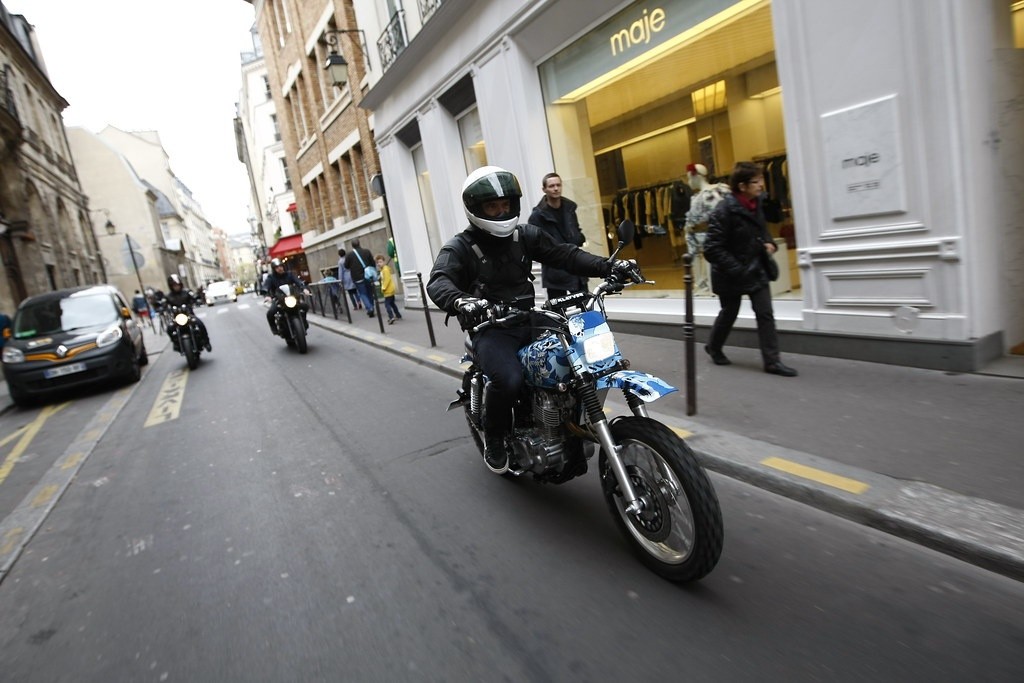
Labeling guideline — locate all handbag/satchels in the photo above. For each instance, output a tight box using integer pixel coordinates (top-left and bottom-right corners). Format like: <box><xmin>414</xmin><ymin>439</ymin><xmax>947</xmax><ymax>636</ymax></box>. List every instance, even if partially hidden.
<box><xmin>364</xmin><ymin>266</ymin><xmax>377</xmax><ymax>282</ymax></box>
<box><xmin>768</xmin><ymin>257</ymin><xmax>778</xmax><ymax>281</ymax></box>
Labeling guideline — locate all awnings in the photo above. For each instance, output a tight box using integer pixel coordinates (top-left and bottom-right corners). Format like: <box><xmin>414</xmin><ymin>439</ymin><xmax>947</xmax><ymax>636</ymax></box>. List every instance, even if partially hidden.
<box><xmin>267</xmin><ymin>233</ymin><xmax>304</xmax><ymax>260</ymax></box>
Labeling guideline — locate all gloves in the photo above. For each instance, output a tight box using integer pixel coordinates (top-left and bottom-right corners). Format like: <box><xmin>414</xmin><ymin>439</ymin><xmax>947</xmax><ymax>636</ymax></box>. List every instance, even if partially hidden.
<box><xmin>454</xmin><ymin>297</ymin><xmax>496</xmax><ymax>329</ymax></box>
<box><xmin>610</xmin><ymin>258</ymin><xmax>647</xmax><ymax>285</ymax></box>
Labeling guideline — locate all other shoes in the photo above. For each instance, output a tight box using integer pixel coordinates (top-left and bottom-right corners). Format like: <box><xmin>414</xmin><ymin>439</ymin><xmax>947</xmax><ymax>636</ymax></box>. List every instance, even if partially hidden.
<box><xmin>359</xmin><ymin>304</ymin><xmax>362</xmax><ymax>309</ymax></box>
<box><xmin>205</xmin><ymin>343</ymin><xmax>211</xmax><ymax>351</ymax></box>
<box><xmin>395</xmin><ymin>317</ymin><xmax>401</xmax><ymax>321</ymax></box>
<box><xmin>367</xmin><ymin>311</ymin><xmax>374</xmax><ymax>317</ymax></box>
<box><xmin>704</xmin><ymin>345</ymin><xmax>731</xmax><ymax>365</ymax></box>
<box><xmin>388</xmin><ymin>317</ymin><xmax>395</xmax><ymax>324</ymax></box>
<box><xmin>174</xmin><ymin>344</ymin><xmax>179</xmax><ymax>351</ymax></box>
<box><xmin>354</xmin><ymin>307</ymin><xmax>357</xmax><ymax>310</ymax></box>
<box><xmin>763</xmin><ymin>360</ymin><xmax>798</xmax><ymax>377</ymax></box>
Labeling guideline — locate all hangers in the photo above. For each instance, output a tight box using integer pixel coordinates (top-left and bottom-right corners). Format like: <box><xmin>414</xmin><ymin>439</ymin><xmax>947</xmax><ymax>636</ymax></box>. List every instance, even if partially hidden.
<box><xmin>612</xmin><ymin>178</ymin><xmax>684</xmax><ymax>199</ymax></box>
<box><xmin>753</xmin><ymin>147</ymin><xmax>787</xmax><ymax>164</ymax></box>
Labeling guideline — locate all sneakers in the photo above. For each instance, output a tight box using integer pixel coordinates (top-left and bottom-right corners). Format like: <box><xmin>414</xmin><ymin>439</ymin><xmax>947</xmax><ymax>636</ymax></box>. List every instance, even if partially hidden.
<box><xmin>483</xmin><ymin>432</ymin><xmax>509</xmax><ymax>474</ymax></box>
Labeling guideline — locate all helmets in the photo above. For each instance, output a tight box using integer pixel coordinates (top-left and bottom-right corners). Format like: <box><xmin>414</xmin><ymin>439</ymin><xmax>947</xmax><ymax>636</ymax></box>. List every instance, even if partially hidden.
<box><xmin>167</xmin><ymin>274</ymin><xmax>183</xmax><ymax>292</ymax></box>
<box><xmin>271</xmin><ymin>258</ymin><xmax>285</xmax><ymax>275</ymax></box>
<box><xmin>461</xmin><ymin>166</ymin><xmax>523</xmax><ymax>237</ymax></box>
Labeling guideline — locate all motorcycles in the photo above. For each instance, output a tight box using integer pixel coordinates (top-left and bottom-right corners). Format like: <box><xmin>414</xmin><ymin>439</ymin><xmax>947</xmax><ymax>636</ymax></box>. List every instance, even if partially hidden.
<box><xmin>152</xmin><ymin>290</ymin><xmax>205</xmax><ymax>370</ymax></box>
<box><xmin>203</xmin><ymin>280</ymin><xmax>238</xmax><ymax>308</ymax></box>
<box><xmin>259</xmin><ymin>275</ymin><xmax>313</xmax><ymax>354</ymax></box>
<box><xmin>444</xmin><ymin>219</ymin><xmax>725</xmax><ymax>586</ymax></box>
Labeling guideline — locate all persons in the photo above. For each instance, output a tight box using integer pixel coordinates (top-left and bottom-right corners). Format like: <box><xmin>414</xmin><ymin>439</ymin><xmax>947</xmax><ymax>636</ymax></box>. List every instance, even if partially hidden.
<box><xmin>261</xmin><ymin>258</ymin><xmax>309</xmax><ymax>335</ymax></box>
<box><xmin>338</xmin><ymin>248</ymin><xmax>362</xmax><ymax>310</ymax></box>
<box><xmin>374</xmin><ymin>254</ymin><xmax>402</xmax><ymax>324</ymax></box>
<box><xmin>527</xmin><ymin>173</ymin><xmax>589</xmax><ymax>317</ymax></box>
<box><xmin>298</xmin><ymin>265</ymin><xmax>312</xmax><ymax>286</ymax></box>
<box><xmin>159</xmin><ymin>274</ymin><xmax>211</xmax><ymax>352</ymax></box>
<box><xmin>323</xmin><ymin>269</ymin><xmax>343</xmax><ymax>314</ymax></box>
<box><xmin>344</xmin><ymin>238</ymin><xmax>375</xmax><ymax>318</ymax></box>
<box><xmin>703</xmin><ymin>162</ymin><xmax>798</xmax><ymax>378</ymax></box>
<box><xmin>427</xmin><ymin>166</ymin><xmax>646</xmax><ymax>473</ymax></box>
<box><xmin>684</xmin><ymin>163</ymin><xmax>731</xmax><ymax>254</ymax></box>
<box><xmin>131</xmin><ymin>285</ymin><xmax>154</xmax><ymax>330</ymax></box>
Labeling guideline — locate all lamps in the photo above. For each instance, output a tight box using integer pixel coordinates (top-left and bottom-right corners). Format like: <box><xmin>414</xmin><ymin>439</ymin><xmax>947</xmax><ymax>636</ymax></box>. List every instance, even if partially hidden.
<box><xmin>91</xmin><ymin>208</ymin><xmax>115</xmax><ymax>236</ymax></box>
<box><xmin>322</xmin><ymin>30</ymin><xmax>372</xmax><ymax>90</ymax></box>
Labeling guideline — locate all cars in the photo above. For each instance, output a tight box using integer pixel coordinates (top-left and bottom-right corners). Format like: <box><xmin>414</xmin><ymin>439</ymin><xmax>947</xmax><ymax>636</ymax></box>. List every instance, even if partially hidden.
<box><xmin>230</xmin><ymin>279</ymin><xmax>244</xmax><ymax>295</ymax></box>
<box><xmin>0</xmin><ymin>284</ymin><xmax>149</xmax><ymax>411</ymax></box>
<box><xmin>243</xmin><ymin>279</ymin><xmax>256</xmax><ymax>294</ymax></box>
